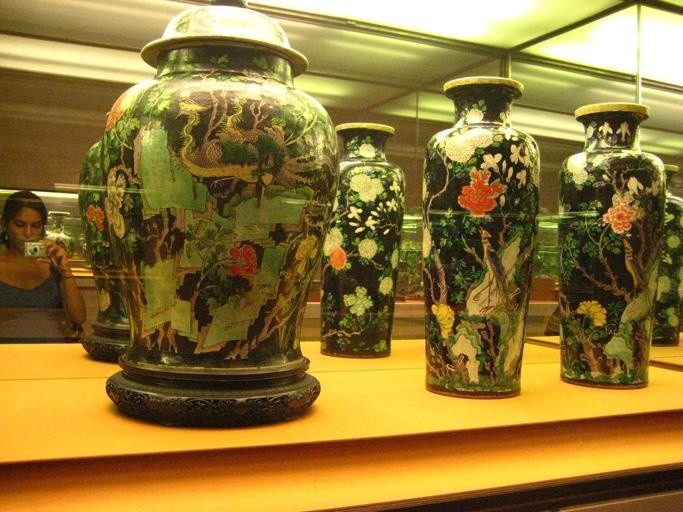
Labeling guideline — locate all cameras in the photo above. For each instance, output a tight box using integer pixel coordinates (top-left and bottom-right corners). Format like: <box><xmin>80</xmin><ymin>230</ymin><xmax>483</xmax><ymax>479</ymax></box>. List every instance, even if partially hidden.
<box><xmin>23</xmin><ymin>241</ymin><xmax>49</xmax><ymax>259</ymax></box>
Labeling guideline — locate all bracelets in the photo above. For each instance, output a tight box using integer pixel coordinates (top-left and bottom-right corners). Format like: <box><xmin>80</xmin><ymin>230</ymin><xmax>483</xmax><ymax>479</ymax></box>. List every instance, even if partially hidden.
<box><xmin>61</xmin><ymin>276</ymin><xmax>74</xmax><ymax>280</ymax></box>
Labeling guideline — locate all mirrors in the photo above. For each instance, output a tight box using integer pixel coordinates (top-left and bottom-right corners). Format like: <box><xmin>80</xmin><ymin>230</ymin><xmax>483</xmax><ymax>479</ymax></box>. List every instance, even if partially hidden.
<box><xmin>508</xmin><ymin>52</ymin><xmax>683</xmax><ymax>373</ymax></box>
<box><xmin>0</xmin><ymin>0</ymin><xmax>507</xmax><ymax>381</ymax></box>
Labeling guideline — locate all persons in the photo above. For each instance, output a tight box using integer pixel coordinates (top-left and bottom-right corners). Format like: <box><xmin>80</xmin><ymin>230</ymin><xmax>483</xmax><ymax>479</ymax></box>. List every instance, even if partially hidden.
<box><xmin>0</xmin><ymin>191</ymin><xmax>87</xmax><ymax>343</ymax></box>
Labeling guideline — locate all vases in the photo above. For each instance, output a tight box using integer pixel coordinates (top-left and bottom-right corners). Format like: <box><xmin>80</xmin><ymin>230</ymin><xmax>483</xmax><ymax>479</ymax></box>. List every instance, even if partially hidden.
<box><xmin>421</xmin><ymin>76</ymin><xmax>540</xmax><ymax>399</ymax></box>
<box><xmin>321</xmin><ymin>122</ymin><xmax>405</xmax><ymax>359</ymax></box>
<box><xmin>652</xmin><ymin>164</ymin><xmax>683</xmax><ymax>347</ymax></box>
<box><xmin>557</xmin><ymin>104</ymin><xmax>667</xmax><ymax>389</ymax></box>
